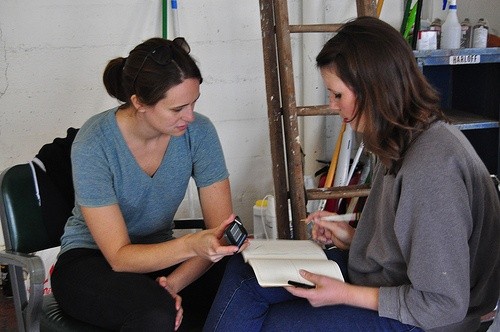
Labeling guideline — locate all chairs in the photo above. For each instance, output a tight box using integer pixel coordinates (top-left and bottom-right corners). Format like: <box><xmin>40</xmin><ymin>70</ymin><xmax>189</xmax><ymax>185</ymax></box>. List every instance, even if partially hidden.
<box><xmin>0</xmin><ymin>127</ymin><xmax>233</xmax><ymax>332</ymax></box>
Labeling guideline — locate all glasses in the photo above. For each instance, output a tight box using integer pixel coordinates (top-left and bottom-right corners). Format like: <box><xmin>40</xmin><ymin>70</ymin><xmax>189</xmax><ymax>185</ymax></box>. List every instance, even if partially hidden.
<box><xmin>132</xmin><ymin>37</ymin><xmax>190</xmax><ymax>96</ymax></box>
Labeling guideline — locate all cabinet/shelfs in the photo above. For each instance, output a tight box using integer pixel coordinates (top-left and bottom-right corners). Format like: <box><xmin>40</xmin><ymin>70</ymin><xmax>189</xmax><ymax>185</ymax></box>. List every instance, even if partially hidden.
<box><xmin>413</xmin><ymin>46</ymin><xmax>500</xmax><ymax>192</ymax></box>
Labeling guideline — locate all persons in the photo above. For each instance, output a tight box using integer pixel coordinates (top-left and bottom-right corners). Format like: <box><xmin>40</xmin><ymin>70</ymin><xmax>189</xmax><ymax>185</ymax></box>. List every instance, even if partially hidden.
<box><xmin>51</xmin><ymin>37</ymin><xmax>250</xmax><ymax>332</ymax></box>
<box><xmin>203</xmin><ymin>15</ymin><xmax>500</xmax><ymax>332</ymax></box>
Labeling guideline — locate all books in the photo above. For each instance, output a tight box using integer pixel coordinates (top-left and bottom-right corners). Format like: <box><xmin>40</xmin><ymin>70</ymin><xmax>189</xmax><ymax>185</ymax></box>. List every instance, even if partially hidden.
<box><xmin>241</xmin><ymin>239</ymin><xmax>344</xmax><ymax>287</ymax></box>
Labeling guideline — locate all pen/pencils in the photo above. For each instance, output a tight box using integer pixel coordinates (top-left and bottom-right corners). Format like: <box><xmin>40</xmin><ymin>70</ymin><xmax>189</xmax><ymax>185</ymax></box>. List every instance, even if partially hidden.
<box><xmin>300</xmin><ymin>213</ymin><xmax>361</xmax><ymax>221</ymax></box>
<box><xmin>288</xmin><ymin>280</ymin><xmax>315</xmax><ymax>289</ymax></box>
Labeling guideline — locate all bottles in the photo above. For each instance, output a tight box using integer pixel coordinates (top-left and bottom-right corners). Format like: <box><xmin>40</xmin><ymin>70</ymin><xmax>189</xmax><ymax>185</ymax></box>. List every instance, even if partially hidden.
<box><xmin>253</xmin><ymin>200</ymin><xmax>268</xmax><ymax>239</ymax></box>
<box><xmin>461</xmin><ymin>18</ymin><xmax>472</xmax><ymax>48</ymax></box>
<box><xmin>472</xmin><ymin>18</ymin><xmax>488</xmax><ymax>48</ymax></box>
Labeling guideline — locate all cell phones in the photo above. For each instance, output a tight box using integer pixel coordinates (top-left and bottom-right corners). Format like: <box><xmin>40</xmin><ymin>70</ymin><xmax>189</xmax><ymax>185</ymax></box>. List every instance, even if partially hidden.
<box><xmin>224</xmin><ymin>216</ymin><xmax>247</xmax><ymax>249</ymax></box>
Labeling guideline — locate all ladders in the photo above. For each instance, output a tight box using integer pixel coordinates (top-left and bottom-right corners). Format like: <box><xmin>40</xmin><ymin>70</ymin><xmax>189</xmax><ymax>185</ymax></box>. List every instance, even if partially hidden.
<box><xmin>261</xmin><ymin>1</ymin><xmax>377</xmax><ymax>240</ymax></box>
